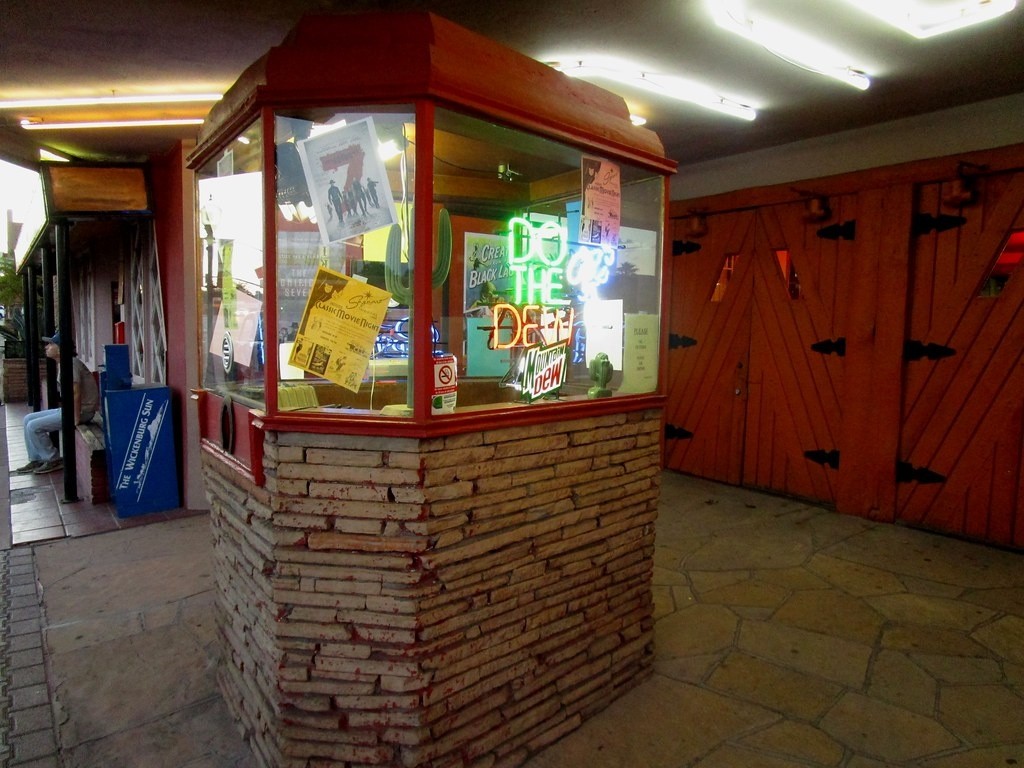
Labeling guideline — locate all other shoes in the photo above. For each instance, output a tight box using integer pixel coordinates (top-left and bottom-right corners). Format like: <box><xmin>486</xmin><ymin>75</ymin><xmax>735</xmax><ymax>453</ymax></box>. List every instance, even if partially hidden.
<box><xmin>329</xmin><ymin>204</ymin><xmax>381</xmax><ymax>222</ymax></box>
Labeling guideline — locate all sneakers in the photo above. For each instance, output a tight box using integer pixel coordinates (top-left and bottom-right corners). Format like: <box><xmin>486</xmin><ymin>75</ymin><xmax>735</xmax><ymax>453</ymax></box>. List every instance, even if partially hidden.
<box><xmin>17</xmin><ymin>457</ymin><xmax>66</xmax><ymax>474</ymax></box>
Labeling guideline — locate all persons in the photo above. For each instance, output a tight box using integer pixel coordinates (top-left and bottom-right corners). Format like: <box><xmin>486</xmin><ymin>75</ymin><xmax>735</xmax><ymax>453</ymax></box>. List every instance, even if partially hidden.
<box><xmin>469</xmin><ymin>247</ymin><xmax>492</xmax><ymax>269</ymax></box>
<box><xmin>14</xmin><ymin>331</ymin><xmax>99</xmax><ymax>475</ymax></box>
<box><xmin>289</xmin><ymin>321</ymin><xmax>301</xmax><ymax>342</ymax></box>
<box><xmin>328</xmin><ymin>180</ymin><xmax>344</xmax><ymax>223</ymax></box>
<box><xmin>470</xmin><ymin>283</ymin><xmax>511</xmax><ymax>310</ymax></box>
<box><xmin>279</xmin><ymin>327</ymin><xmax>288</xmax><ymax>342</ymax></box>
<box><xmin>341</xmin><ymin>177</ymin><xmax>375</xmax><ymax>217</ymax></box>
<box><xmin>367</xmin><ymin>177</ymin><xmax>381</xmax><ymax>208</ymax></box>
<box><xmin>326</xmin><ymin>202</ymin><xmax>334</xmax><ymax>218</ymax></box>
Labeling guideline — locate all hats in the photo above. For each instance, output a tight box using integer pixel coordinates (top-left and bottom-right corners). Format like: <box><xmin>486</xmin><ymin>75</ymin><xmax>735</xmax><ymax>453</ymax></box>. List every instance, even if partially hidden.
<box><xmin>43</xmin><ymin>333</ymin><xmax>60</xmax><ymax>347</ymax></box>
<box><xmin>329</xmin><ymin>180</ymin><xmax>336</xmax><ymax>184</ymax></box>
